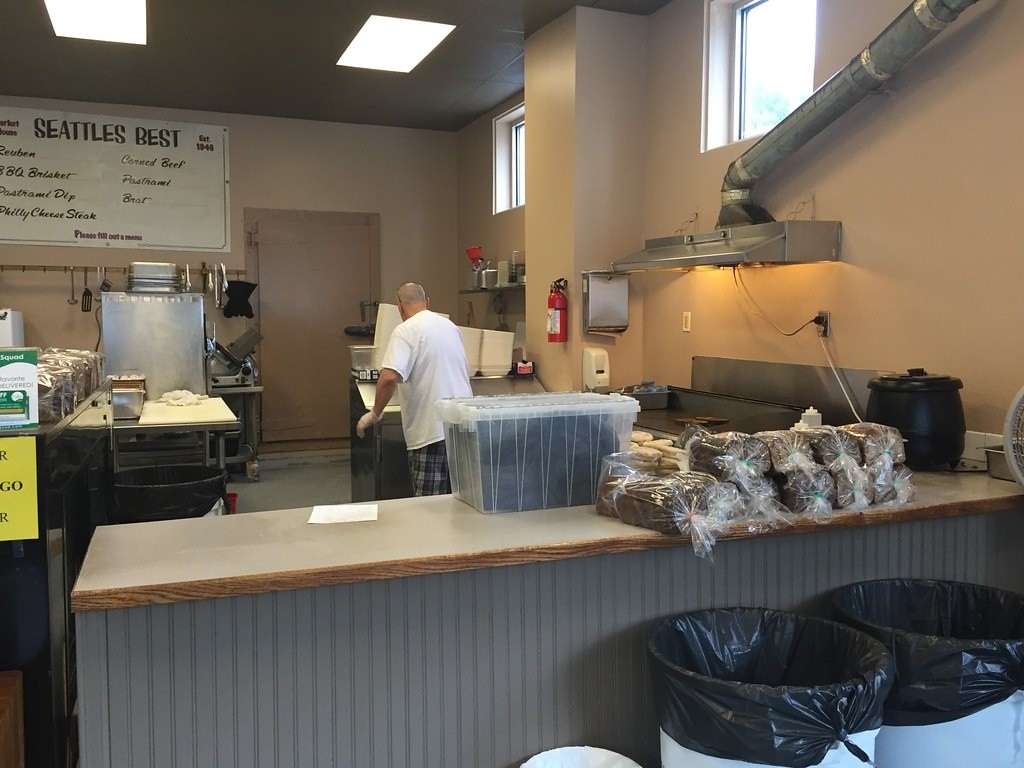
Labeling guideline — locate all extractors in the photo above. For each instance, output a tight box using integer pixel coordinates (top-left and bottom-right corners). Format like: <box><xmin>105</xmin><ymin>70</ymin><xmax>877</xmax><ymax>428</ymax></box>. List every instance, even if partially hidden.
<box><xmin>611</xmin><ymin>0</ymin><xmax>984</xmax><ymax>273</ymax></box>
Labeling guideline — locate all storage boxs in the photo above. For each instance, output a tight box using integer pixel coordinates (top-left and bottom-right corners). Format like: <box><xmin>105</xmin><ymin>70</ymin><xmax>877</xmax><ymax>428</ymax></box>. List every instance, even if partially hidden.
<box><xmin>437</xmin><ymin>388</ymin><xmax>641</xmax><ymax>516</ymax></box>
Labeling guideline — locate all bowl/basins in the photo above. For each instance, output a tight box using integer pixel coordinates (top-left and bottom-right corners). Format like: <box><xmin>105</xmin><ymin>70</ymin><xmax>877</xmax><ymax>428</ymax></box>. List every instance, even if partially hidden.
<box><xmin>617</xmin><ymin>391</ymin><xmax>671</xmax><ymax>410</ymax></box>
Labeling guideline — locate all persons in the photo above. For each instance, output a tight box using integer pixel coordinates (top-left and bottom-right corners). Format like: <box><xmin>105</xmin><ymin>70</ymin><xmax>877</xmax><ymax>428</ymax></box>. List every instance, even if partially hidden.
<box><xmin>355</xmin><ymin>282</ymin><xmax>474</xmax><ymax>497</ymax></box>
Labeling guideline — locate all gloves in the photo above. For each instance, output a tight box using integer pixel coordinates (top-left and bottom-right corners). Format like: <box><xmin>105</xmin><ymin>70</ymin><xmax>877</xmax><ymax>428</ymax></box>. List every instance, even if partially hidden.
<box><xmin>356</xmin><ymin>407</ymin><xmax>384</xmax><ymax>439</ymax></box>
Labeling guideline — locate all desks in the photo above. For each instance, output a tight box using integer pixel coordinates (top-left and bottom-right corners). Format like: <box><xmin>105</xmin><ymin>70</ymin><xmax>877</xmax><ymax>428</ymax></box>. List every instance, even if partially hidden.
<box><xmin>69</xmin><ymin>449</ymin><xmax>1024</xmax><ymax>767</ymax></box>
<box><xmin>72</xmin><ymin>391</ymin><xmax>242</xmax><ymax>490</ymax></box>
<box><xmin>210</xmin><ymin>382</ymin><xmax>263</xmax><ymax>477</ymax></box>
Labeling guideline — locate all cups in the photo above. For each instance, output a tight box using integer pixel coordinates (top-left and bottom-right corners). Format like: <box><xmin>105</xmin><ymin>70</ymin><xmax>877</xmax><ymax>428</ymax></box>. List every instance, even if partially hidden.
<box><xmin>485</xmin><ymin>270</ymin><xmax>498</xmax><ymax>289</ymax></box>
<box><xmin>516</xmin><ymin>267</ymin><xmax>525</xmax><ymax>286</ymax></box>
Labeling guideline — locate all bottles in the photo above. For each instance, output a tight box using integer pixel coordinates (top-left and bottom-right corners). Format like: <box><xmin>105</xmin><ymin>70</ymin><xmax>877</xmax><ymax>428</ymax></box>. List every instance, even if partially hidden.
<box><xmin>801</xmin><ymin>406</ymin><xmax>822</xmax><ymax>427</ymax></box>
<box><xmin>791</xmin><ymin>420</ymin><xmax>811</xmax><ymax>431</ymax></box>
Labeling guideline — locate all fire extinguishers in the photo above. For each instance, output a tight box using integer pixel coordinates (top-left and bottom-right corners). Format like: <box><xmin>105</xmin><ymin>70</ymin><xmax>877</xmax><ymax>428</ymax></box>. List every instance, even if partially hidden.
<box><xmin>546</xmin><ymin>278</ymin><xmax>569</xmax><ymax>343</ymax></box>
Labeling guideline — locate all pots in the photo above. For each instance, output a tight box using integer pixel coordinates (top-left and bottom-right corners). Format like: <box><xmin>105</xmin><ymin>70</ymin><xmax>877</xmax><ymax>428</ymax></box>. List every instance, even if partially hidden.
<box><xmin>864</xmin><ymin>368</ymin><xmax>967</xmax><ymax>472</ymax></box>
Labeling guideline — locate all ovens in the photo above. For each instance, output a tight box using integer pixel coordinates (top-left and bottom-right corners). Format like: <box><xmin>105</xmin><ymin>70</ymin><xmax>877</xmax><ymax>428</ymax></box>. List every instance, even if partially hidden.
<box><xmin>99</xmin><ymin>292</ymin><xmax>212</xmax><ymax>398</ymax></box>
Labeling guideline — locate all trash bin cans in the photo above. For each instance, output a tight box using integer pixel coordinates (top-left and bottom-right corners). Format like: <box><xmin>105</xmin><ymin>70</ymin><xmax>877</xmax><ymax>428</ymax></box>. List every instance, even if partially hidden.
<box><xmin>649</xmin><ymin>606</ymin><xmax>896</xmax><ymax>768</ymax></box>
<box><xmin>834</xmin><ymin>575</ymin><xmax>1023</xmax><ymax>768</ymax></box>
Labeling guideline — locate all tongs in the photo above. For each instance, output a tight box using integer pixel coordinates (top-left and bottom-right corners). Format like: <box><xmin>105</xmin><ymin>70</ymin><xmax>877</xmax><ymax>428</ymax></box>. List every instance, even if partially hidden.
<box><xmin>214</xmin><ymin>264</ymin><xmax>220</xmax><ymax>309</ymax></box>
<box><xmin>220</xmin><ymin>262</ymin><xmax>229</xmax><ymax>291</ymax></box>
<box><xmin>208</xmin><ymin>265</ymin><xmax>214</xmax><ymax>292</ymax></box>
<box><xmin>185</xmin><ymin>263</ymin><xmax>191</xmax><ymax>293</ymax></box>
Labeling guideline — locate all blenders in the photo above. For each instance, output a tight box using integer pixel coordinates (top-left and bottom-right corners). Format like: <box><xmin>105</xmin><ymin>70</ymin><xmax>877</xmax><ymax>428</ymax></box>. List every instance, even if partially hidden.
<box><xmin>210</xmin><ymin>325</ymin><xmax>264</xmax><ymax>386</ymax></box>
